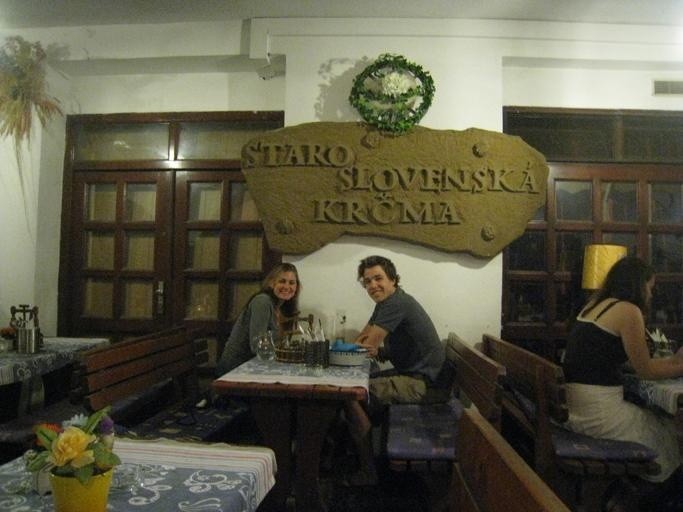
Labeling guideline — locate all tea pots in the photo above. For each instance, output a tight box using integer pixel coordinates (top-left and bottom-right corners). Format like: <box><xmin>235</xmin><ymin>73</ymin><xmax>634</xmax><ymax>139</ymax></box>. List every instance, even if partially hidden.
<box><xmin>251</xmin><ymin>327</ymin><xmax>276</xmax><ymax>363</ymax></box>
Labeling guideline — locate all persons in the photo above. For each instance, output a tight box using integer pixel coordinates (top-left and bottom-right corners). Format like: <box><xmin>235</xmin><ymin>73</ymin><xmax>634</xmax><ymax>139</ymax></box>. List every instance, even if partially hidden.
<box><xmin>213</xmin><ymin>263</ymin><xmax>301</xmax><ymax>404</ymax></box>
<box><xmin>337</xmin><ymin>255</ymin><xmax>452</xmax><ymax>490</ymax></box>
<box><xmin>549</xmin><ymin>254</ymin><xmax>683</xmax><ymax>510</ymax></box>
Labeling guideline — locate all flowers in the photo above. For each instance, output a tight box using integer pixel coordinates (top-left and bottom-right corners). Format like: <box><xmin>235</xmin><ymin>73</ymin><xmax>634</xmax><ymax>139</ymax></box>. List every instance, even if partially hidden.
<box><xmin>350</xmin><ymin>52</ymin><xmax>435</xmax><ymax>132</ymax></box>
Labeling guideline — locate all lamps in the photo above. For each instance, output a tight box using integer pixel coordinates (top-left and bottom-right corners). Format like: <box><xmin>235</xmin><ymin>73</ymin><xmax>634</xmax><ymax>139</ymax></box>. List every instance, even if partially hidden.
<box><xmin>581</xmin><ymin>244</ymin><xmax>628</xmax><ymax>290</ymax></box>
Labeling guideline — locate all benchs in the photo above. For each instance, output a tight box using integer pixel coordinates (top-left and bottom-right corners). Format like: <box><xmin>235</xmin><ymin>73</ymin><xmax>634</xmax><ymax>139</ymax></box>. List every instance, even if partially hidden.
<box><xmin>0</xmin><ymin>325</ymin><xmax>659</xmax><ymax>512</ymax></box>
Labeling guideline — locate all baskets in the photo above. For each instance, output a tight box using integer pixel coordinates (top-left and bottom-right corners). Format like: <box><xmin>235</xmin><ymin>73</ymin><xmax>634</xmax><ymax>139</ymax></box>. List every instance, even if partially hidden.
<box><xmin>275</xmin><ymin>340</ymin><xmax>368</xmax><ymax>369</ymax></box>
